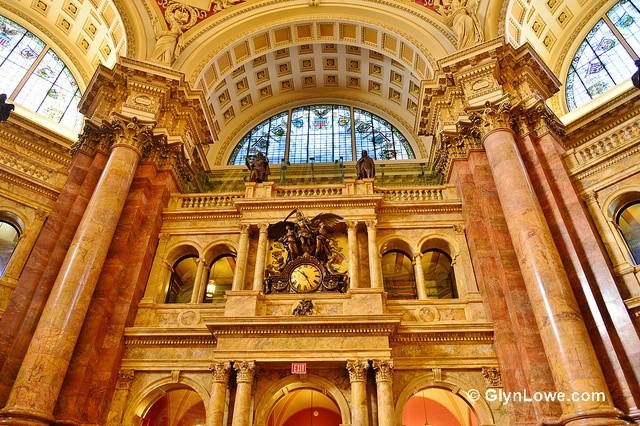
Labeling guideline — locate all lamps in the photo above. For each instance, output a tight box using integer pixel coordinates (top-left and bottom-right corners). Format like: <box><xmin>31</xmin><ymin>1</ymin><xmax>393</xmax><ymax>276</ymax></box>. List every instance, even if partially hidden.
<box><xmin>205</xmin><ymin>262</ymin><xmax>217</xmax><ymax>298</ymax></box>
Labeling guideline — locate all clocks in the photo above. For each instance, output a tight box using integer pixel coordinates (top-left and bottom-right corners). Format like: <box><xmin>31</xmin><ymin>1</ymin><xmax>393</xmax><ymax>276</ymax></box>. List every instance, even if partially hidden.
<box><xmin>288</xmin><ymin>262</ymin><xmax>323</xmax><ymax>294</ymax></box>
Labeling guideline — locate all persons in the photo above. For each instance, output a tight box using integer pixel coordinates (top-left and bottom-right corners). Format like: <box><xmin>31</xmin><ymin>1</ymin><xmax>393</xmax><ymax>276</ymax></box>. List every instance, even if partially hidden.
<box><xmin>279</xmin><ymin>212</ymin><xmax>334</xmax><ymax>261</ymax></box>
<box><xmin>355</xmin><ymin>150</ymin><xmax>375</xmax><ymax>179</ymax></box>
<box><xmin>246</xmin><ymin>152</ymin><xmax>271</xmax><ymax>182</ymax></box>
